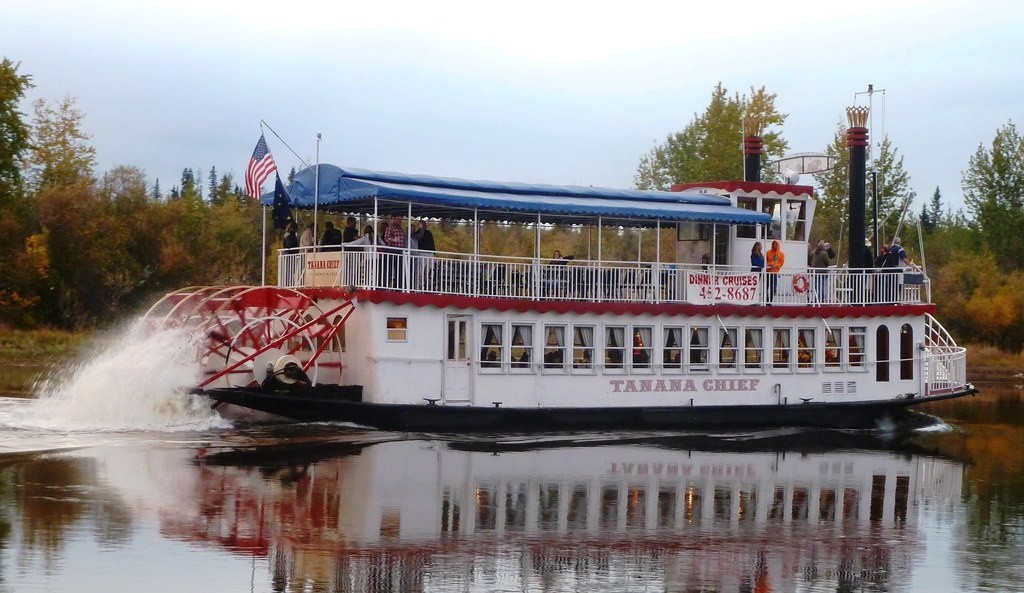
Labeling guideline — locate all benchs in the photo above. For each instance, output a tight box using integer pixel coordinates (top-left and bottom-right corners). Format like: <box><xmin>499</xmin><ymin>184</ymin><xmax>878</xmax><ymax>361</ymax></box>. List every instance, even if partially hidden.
<box><xmin>430</xmin><ymin>262</ymin><xmax>488</xmax><ymax>293</ymax></box>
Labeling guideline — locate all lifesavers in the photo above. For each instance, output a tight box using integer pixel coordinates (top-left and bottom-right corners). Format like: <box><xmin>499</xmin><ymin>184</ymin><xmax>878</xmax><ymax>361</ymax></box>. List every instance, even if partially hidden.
<box><xmin>792</xmin><ymin>274</ymin><xmax>810</xmax><ymax>293</ymax></box>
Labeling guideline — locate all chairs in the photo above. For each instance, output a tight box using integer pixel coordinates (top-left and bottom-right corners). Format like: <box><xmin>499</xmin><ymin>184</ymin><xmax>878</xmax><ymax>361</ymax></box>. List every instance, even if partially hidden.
<box><xmin>544</xmin><ymin>350</ymin><xmax>563</xmax><ymax>368</ymax></box>
<box><xmin>546</xmin><ymin>270</ymin><xmax>560</xmax><ymax>296</ymax></box>
<box><xmin>530</xmin><ymin>269</ymin><xmax>548</xmax><ymax>296</ymax></box>
<box><xmin>492</xmin><ymin>264</ymin><xmax>508</xmax><ymax>293</ymax></box>
<box><xmin>508</xmin><ymin>271</ymin><xmax>525</xmax><ymax>294</ymax></box>
<box><xmin>582</xmin><ymin>269</ymin><xmax>600</xmax><ymax>297</ymax></box>
<box><xmin>559</xmin><ymin>270</ymin><xmax>572</xmax><ymax>296</ymax></box>
<box><xmin>485</xmin><ymin>352</ymin><xmax>497</xmax><ymax>368</ymax></box>
<box><xmin>623</xmin><ymin>270</ymin><xmax>637</xmax><ymax>297</ymax></box>
<box><xmin>568</xmin><ymin>269</ymin><xmax>585</xmax><ymax>298</ymax></box>
<box><xmin>638</xmin><ymin>271</ymin><xmax>652</xmax><ymax>299</ymax></box>
<box><xmin>653</xmin><ymin>271</ymin><xmax>669</xmax><ymax>299</ymax></box>
<box><xmin>519</xmin><ymin>352</ymin><xmax>529</xmax><ymax>368</ymax></box>
<box><xmin>607</xmin><ymin>269</ymin><xmax>620</xmax><ymax>298</ymax></box>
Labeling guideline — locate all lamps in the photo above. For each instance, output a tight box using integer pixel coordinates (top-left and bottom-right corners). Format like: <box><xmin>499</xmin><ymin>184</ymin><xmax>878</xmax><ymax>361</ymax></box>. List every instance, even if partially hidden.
<box><xmin>917</xmin><ymin>342</ymin><xmax>926</xmax><ymax>352</ymax></box>
<box><xmin>346</xmin><ymin>285</ymin><xmax>362</xmax><ymax>296</ymax></box>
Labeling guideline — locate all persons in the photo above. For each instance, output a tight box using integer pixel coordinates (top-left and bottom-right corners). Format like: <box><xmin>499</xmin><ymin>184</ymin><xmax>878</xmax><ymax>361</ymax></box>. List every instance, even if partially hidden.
<box><xmin>485</xmin><ymin>349</ymin><xmax>591</xmax><ymax>368</ymax></box>
<box><xmin>807</xmin><ymin>239</ymin><xmax>840</xmax><ymax>306</ymax></box>
<box><xmin>750</xmin><ymin>239</ymin><xmax>785</xmax><ymax>305</ymax></box>
<box><xmin>864</xmin><ymin>235</ymin><xmax>916</xmax><ymax>305</ymax></box>
<box><xmin>282</xmin><ymin>216</ymin><xmax>437</xmax><ymax>293</ymax></box>
<box><xmin>542</xmin><ymin>249</ymin><xmax>574</xmax><ymax>299</ymax></box>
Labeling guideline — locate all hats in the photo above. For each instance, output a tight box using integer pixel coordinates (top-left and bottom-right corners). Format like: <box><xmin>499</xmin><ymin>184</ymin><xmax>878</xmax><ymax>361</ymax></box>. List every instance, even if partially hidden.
<box><xmin>883</xmin><ymin>245</ymin><xmax>890</xmax><ymax>252</ymax></box>
<box><xmin>865</xmin><ymin>238</ymin><xmax>872</xmax><ymax>247</ymax></box>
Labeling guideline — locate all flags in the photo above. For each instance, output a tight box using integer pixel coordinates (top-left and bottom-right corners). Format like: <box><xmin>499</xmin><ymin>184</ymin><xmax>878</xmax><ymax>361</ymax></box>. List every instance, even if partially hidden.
<box><xmin>271</xmin><ymin>170</ymin><xmax>294</xmax><ymax>228</ymax></box>
<box><xmin>245</xmin><ymin>131</ymin><xmax>278</xmax><ymax>200</ymax></box>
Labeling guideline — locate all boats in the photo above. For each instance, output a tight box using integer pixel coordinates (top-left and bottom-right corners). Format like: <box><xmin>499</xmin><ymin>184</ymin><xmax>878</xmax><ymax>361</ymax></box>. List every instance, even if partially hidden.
<box><xmin>162</xmin><ymin>427</ymin><xmax>975</xmax><ymax>592</ymax></box>
<box><xmin>136</xmin><ymin>82</ymin><xmax>981</xmax><ymax>434</ymax></box>
